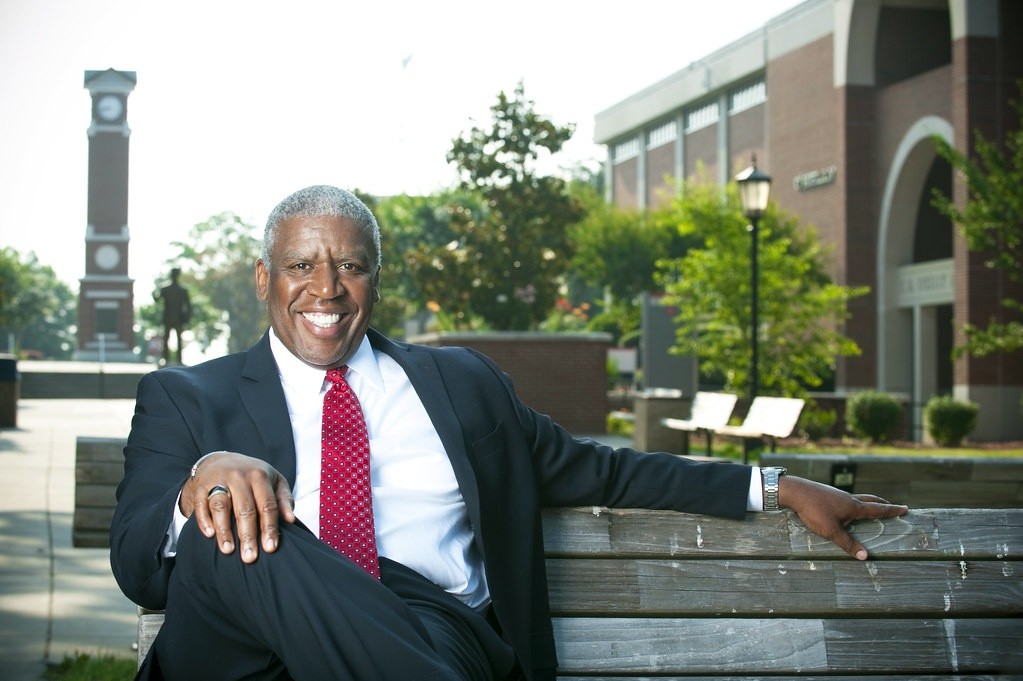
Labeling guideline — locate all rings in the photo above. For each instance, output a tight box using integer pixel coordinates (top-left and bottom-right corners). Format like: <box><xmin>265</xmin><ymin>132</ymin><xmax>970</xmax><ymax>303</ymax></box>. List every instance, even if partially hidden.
<box><xmin>207</xmin><ymin>485</ymin><xmax>231</xmax><ymax>501</ymax></box>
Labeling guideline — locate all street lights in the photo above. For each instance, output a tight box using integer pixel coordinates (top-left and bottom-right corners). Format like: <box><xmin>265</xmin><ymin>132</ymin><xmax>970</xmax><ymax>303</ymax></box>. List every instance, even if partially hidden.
<box><xmin>733</xmin><ymin>153</ymin><xmax>773</xmax><ymax>449</ymax></box>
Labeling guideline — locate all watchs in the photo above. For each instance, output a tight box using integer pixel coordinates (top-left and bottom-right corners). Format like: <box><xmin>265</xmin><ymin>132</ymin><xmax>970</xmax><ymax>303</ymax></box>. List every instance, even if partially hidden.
<box><xmin>761</xmin><ymin>466</ymin><xmax>787</xmax><ymax>511</ymax></box>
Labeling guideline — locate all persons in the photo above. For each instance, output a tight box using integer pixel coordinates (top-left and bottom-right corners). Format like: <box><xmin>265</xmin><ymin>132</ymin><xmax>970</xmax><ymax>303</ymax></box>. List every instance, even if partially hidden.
<box><xmin>152</xmin><ymin>268</ymin><xmax>192</xmax><ymax>369</ymax></box>
<box><xmin>108</xmin><ymin>186</ymin><xmax>910</xmax><ymax>681</ymax></box>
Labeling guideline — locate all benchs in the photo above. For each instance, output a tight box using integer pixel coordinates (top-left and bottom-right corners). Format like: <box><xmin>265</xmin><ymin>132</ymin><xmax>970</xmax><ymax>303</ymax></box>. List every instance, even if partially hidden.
<box><xmin>661</xmin><ymin>392</ymin><xmax>738</xmax><ymax>458</ymax></box>
<box><xmin>713</xmin><ymin>395</ymin><xmax>807</xmax><ymax>467</ymax></box>
<box><xmin>71</xmin><ymin>438</ymin><xmax>1023</xmax><ymax>681</ymax></box>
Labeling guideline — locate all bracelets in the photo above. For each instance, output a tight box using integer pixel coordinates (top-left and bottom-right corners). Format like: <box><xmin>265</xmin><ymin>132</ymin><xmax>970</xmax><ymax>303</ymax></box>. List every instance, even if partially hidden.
<box><xmin>190</xmin><ymin>450</ymin><xmax>227</xmax><ymax>480</ymax></box>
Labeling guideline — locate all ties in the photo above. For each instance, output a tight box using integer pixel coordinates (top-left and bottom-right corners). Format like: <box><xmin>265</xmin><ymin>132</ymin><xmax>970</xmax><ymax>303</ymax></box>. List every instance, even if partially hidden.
<box><xmin>318</xmin><ymin>365</ymin><xmax>378</xmax><ymax>580</ymax></box>
